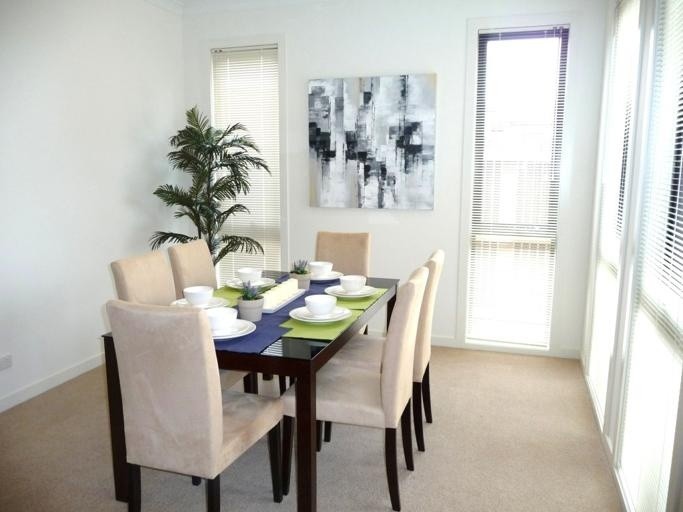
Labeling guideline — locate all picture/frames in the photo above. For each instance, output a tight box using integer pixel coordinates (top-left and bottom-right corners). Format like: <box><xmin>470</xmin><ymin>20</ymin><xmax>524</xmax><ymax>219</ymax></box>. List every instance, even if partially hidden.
<box><xmin>305</xmin><ymin>73</ymin><xmax>437</xmax><ymax>211</ymax></box>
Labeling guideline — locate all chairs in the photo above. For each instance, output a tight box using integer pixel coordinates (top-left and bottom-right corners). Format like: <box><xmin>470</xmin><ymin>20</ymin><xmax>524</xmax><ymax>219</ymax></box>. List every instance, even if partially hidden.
<box><xmin>313</xmin><ymin>231</ymin><xmax>371</xmax><ymax>277</ymax></box>
<box><xmin>167</xmin><ymin>239</ymin><xmax>286</xmax><ymax>396</ymax></box>
<box><xmin>281</xmin><ymin>267</ymin><xmax>430</xmax><ymax>512</ymax></box>
<box><xmin>110</xmin><ymin>249</ymin><xmax>253</xmax><ymax>395</ymax></box>
<box><xmin>105</xmin><ymin>299</ymin><xmax>288</xmax><ymax>512</ymax></box>
<box><xmin>315</xmin><ymin>248</ymin><xmax>446</xmax><ymax>452</ymax></box>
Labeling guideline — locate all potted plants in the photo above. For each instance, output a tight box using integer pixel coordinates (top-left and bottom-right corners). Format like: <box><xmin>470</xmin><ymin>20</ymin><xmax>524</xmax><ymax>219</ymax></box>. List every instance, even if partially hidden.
<box><xmin>289</xmin><ymin>258</ymin><xmax>313</xmax><ymax>291</ymax></box>
<box><xmin>238</xmin><ymin>281</ymin><xmax>265</xmax><ymax>322</ymax></box>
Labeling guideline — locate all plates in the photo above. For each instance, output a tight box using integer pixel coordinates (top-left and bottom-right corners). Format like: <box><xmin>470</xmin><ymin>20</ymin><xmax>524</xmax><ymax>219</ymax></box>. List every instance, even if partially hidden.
<box><xmin>212</xmin><ymin>318</ymin><xmax>257</xmax><ymax>340</ymax></box>
<box><xmin>295</xmin><ymin>308</ymin><xmax>344</xmax><ymax>320</ymax></box>
<box><xmin>304</xmin><ymin>272</ymin><xmax>343</xmax><ymax>283</ymax></box>
<box><xmin>288</xmin><ymin>306</ymin><xmax>353</xmax><ymax>324</ymax></box>
<box><xmin>324</xmin><ymin>286</ymin><xmax>379</xmax><ymax>300</ymax></box>
<box><xmin>211</xmin><ymin>323</ymin><xmax>250</xmax><ymax>337</ymax></box>
<box><xmin>226</xmin><ymin>278</ymin><xmax>276</xmax><ymax>289</ymax></box>
<box><xmin>176</xmin><ymin>298</ymin><xmax>223</xmax><ymax>307</ymax></box>
<box><xmin>169</xmin><ymin>298</ymin><xmax>230</xmax><ymax>309</ymax></box>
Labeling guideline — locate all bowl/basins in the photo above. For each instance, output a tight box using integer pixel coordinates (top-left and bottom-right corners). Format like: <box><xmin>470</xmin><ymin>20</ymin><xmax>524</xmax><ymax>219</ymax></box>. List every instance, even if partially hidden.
<box><xmin>309</xmin><ymin>262</ymin><xmax>333</xmax><ymax>277</ymax></box>
<box><xmin>340</xmin><ymin>275</ymin><xmax>367</xmax><ymax>292</ymax></box>
<box><xmin>205</xmin><ymin>306</ymin><xmax>238</xmax><ymax>322</ymax></box>
<box><xmin>183</xmin><ymin>286</ymin><xmax>214</xmax><ymax>307</ymax></box>
<box><xmin>238</xmin><ymin>268</ymin><xmax>263</xmax><ymax>282</ymax></box>
<box><xmin>305</xmin><ymin>294</ymin><xmax>338</xmax><ymax>316</ymax></box>
